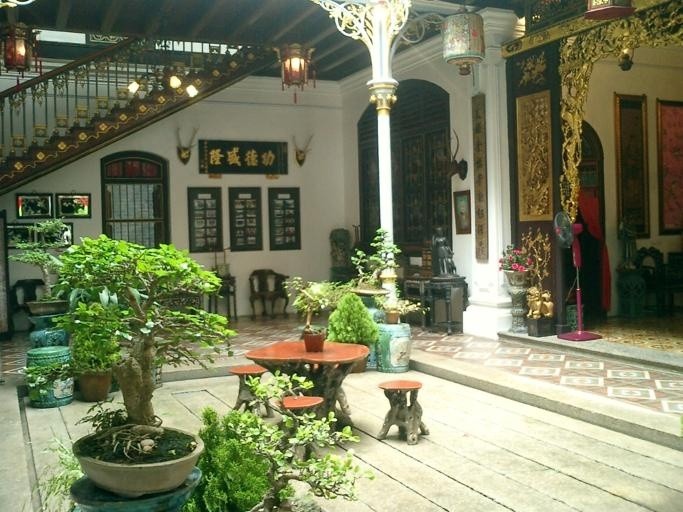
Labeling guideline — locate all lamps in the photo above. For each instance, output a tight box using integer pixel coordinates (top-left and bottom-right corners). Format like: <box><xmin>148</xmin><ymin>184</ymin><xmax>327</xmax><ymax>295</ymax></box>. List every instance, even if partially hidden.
<box><xmin>616</xmin><ymin>46</ymin><xmax>636</xmax><ymax>73</ymax></box>
<box><xmin>272</xmin><ymin>40</ymin><xmax>319</xmax><ymax>106</ymax></box>
<box><xmin>0</xmin><ymin>21</ymin><xmax>44</xmax><ymax>94</ymax></box>
<box><xmin>582</xmin><ymin>0</ymin><xmax>636</xmax><ymax>22</ymax></box>
<box><xmin>123</xmin><ymin>25</ymin><xmax>202</xmax><ymax>100</ymax></box>
<box><xmin>439</xmin><ymin>1</ymin><xmax>487</xmax><ymax>80</ymax></box>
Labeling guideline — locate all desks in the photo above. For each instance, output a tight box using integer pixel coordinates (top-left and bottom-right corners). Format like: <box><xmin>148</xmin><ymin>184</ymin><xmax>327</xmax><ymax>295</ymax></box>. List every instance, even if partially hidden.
<box><xmin>208</xmin><ymin>276</ymin><xmax>238</xmax><ymax>321</ymax></box>
<box><xmin>397</xmin><ymin>270</ymin><xmax>466</xmax><ymax>336</ymax></box>
<box><xmin>245</xmin><ymin>341</ymin><xmax>370</xmax><ymax>419</ymax></box>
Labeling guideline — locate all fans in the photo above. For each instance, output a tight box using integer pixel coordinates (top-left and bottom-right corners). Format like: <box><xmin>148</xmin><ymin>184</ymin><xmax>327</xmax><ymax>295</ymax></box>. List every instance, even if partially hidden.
<box><xmin>553</xmin><ymin>209</ymin><xmax>604</xmax><ymax>343</ymax></box>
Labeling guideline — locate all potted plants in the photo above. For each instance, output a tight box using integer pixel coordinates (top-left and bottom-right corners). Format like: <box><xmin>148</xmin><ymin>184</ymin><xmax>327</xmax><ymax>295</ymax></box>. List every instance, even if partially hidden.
<box><xmin>282</xmin><ymin>277</ymin><xmax>360</xmax><ymax>352</ymax></box>
<box><xmin>351</xmin><ymin>228</ymin><xmax>402</xmax><ymax>290</ymax></box>
<box><xmin>371</xmin><ymin>289</ymin><xmax>431</xmax><ymax>323</ymax></box>
<box><xmin>8</xmin><ymin>222</ymin><xmax>71</xmax><ymax>315</ymax></box>
<box><xmin>50</xmin><ymin>235</ymin><xmax>239</xmax><ymax>497</ymax></box>
<box><xmin>324</xmin><ymin>291</ymin><xmax>379</xmax><ymax>375</ymax></box>
<box><xmin>229</xmin><ymin>371</ymin><xmax>375</xmax><ymax>512</ymax></box>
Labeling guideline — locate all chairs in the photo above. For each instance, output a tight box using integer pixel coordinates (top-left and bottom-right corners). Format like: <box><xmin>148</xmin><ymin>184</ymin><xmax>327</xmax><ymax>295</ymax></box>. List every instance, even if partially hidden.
<box><xmin>631</xmin><ymin>244</ymin><xmax>682</xmax><ymax>320</ymax></box>
<box><xmin>250</xmin><ymin>269</ymin><xmax>289</xmax><ymax>321</ymax></box>
<box><xmin>7</xmin><ymin>279</ymin><xmax>46</xmax><ymax>342</ymax></box>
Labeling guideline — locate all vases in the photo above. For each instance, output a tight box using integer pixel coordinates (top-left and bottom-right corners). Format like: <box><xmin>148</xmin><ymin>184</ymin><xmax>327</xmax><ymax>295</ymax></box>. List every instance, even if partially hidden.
<box><xmin>504</xmin><ymin>267</ymin><xmax>531</xmax><ymax>291</ymax></box>
<box><xmin>27</xmin><ymin>315</ymin><xmax>69</xmax><ymax>348</ymax></box>
<box><xmin>521</xmin><ymin>311</ymin><xmax>557</xmax><ymax>339</ymax></box>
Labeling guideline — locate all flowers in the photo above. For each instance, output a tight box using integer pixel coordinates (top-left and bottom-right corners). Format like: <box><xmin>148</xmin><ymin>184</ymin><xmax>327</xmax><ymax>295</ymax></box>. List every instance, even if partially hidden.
<box><xmin>494</xmin><ymin>239</ymin><xmax>536</xmax><ymax>274</ymax></box>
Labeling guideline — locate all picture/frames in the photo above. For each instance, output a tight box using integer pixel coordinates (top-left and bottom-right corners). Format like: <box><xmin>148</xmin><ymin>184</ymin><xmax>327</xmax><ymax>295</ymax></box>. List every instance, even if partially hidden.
<box><xmin>13</xmin><ymin>191</ymin><xmax>55</xmax><ymax>220</ymax></box>
<box><xmin>6</xmin><ymin>223</ymin><xmax>73</xmax><ymax>249</ymax></box>
<box><xmin>451</xmin><ymin>188</ymin><xmax>472</xmax><ymax>237</ymax></box>
<box><xmin>609</xmin><ymin>90</ymin><xmax>651</xmax><ymax>241</ymax></box>
<box><xmin>652</xmin><ymin>96</ymin><xmax>682</xmax><ymax>239</ymax></box>
<box><xmin>55</xmin><ymin>192</ymin><xmax>92</xmax><ymax>220</ymax></box>
<box><xmin>181</xmin><ymin>181</ymin><xmax>305</xmax><ymax>257</ymax></box>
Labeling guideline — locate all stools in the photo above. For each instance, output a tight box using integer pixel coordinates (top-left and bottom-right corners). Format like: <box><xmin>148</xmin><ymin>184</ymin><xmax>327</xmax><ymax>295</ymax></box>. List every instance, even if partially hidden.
<box><xmin>276</xmin><ymin>396</ymin><xmax>325</xmax><ymax>460</ymax></box>
<box><xmin>376</xmin><ymin>380</ymin><xmax>430</xmax><ymax>445</ymax></box>
<box><xmin>230</xmin><ymin>364</ymin><xmax>275</xmax><ymax>418</ymax></box>
